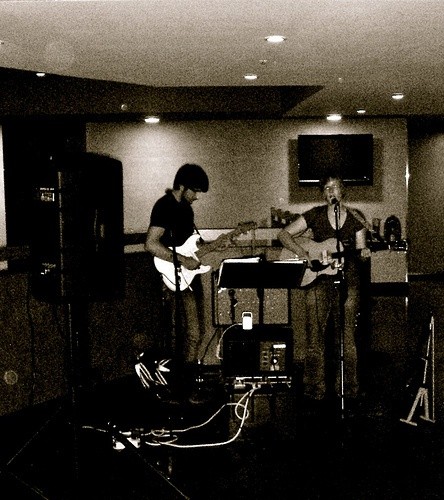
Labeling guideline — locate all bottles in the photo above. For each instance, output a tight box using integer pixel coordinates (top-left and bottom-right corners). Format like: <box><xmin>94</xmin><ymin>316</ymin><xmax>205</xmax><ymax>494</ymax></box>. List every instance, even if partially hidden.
<box><xmin>390</xmin><ymin>227</ymin><xmax>396</xmax><ymax>247</ymax></box>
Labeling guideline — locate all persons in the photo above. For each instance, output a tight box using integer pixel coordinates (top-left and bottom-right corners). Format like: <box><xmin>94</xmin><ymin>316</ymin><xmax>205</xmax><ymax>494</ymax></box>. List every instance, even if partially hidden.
<box><xmin>277</xmin><ymin>173</ymin><xmax>371</xmax><ymax>419</ymax></box>
<box><xmin>144</xmin><ymin>164</ymin><xmax>232</xmax><ymax>405</ymax></box>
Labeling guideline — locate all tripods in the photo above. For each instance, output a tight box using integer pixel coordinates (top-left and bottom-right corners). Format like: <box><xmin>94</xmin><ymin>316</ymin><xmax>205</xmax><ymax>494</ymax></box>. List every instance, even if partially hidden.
<box><xmin>0</xmin><ymin>305</ymin><xmax>192</xmax><ymax>500</ymax></box>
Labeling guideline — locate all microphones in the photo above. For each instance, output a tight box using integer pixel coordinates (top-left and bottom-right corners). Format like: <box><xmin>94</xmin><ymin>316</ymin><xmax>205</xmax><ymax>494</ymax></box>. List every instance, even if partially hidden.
<box><xmin>331</xmin><ymin>197</ymin><xmax>339</xmax><ymax>207</ymax></box>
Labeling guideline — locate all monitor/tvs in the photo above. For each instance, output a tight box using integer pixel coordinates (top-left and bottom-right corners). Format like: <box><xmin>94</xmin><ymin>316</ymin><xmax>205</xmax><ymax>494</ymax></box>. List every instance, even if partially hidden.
<box><xmin>298</xmin><ymin>134</ymin><xmax>374</xmax><ymax>188</ymax></box>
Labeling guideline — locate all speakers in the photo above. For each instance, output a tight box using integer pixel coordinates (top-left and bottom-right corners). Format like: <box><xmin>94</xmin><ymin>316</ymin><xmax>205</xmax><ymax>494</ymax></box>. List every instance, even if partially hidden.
<box><xmin>28</xmin><ymin>151</ymin><xmax>126</xmax><ymax>306</ymax></box>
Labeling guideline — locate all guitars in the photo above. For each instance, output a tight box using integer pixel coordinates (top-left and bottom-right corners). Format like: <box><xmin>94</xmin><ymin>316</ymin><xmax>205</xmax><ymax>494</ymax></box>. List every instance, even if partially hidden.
<box><xmin>280</xmin><ymin>237</ymin><xmax>409</xmax><ymax>290</ymax></box>
<box><xmin>154</xmin><ymin>221</ymin><xmax>258</xmax><ymax>291</ymax></box>
